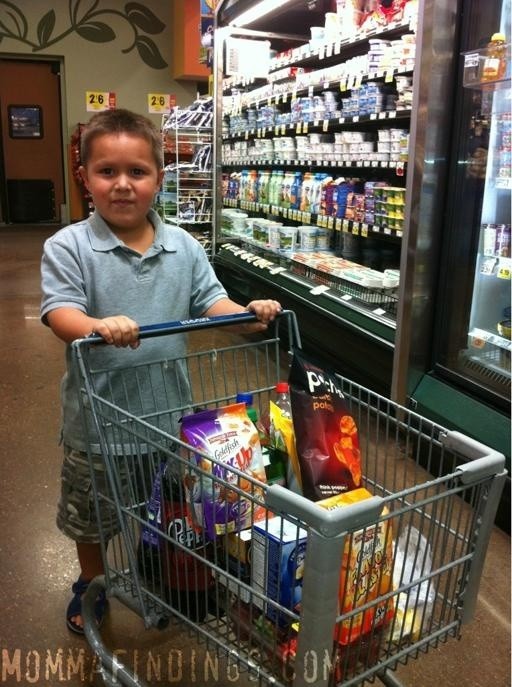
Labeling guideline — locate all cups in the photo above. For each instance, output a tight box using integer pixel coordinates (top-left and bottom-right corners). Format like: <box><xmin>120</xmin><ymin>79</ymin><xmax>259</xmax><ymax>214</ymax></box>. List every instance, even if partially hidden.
<box><xmin>483</xmin><ymin>111</ymin><xmax>511</xmax><ymax>258</ymax></box>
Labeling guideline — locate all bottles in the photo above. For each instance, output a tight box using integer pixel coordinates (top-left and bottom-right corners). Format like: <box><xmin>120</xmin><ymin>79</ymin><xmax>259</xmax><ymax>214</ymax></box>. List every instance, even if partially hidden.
<box><xmin>222</xmin><ymin>170</ymin><xmax>349</xmax><ymax>218</ymax></box>
<box><xmin>235</xmin><ymin>383</ymin><xmax>293</xmax><ymax>445</ymax></box>
<box><xmin>481</xmin><ymin>32</ymin><xmax>507</xmax><ymax>84</ymax></box>
<box><xmin>137</xmin><ymin>439</ymin><xmax>224</xmax><ymax>622</ymax></box>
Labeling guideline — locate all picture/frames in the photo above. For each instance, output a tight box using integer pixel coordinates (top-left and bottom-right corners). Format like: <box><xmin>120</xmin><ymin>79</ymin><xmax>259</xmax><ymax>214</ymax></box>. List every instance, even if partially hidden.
<box><xmin>8</xmin><ymin>105</ymin><xmax>43</xmax><ymax>138</ymax></box>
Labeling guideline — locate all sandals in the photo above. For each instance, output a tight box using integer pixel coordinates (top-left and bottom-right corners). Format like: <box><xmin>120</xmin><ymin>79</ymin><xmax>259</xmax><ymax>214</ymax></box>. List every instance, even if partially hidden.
<box><xmin>66</xmin><ymin>577</ymin><xmax>109</xmax><ymax>633</ymax></box>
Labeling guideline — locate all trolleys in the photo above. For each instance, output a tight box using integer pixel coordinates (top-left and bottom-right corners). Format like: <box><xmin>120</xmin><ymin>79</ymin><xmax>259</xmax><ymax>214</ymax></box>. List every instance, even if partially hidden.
<box><xmin>54</xmin><ymin>307</ymin><xmax>504</xmax><ymax>668</ymax></box>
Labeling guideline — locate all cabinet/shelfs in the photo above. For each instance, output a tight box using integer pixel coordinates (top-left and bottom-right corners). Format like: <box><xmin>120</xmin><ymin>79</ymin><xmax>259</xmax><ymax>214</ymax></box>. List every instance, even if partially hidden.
<box><xmin>70</xmin><ymin>107</ymin><xmax>213</xmax><ymax>266</ymax></box>
<box><xmin>466</xmin><ymin>1</ymin><xmax>510</xmax><ymax>379</ymax></box>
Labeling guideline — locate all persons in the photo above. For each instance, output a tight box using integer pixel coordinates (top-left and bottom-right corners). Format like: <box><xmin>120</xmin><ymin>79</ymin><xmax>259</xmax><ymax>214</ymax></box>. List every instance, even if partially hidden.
<box><xmin>38</xmin><ymin>107</ymin><xmax>283</xmax><ymax>633</ymax></box>
